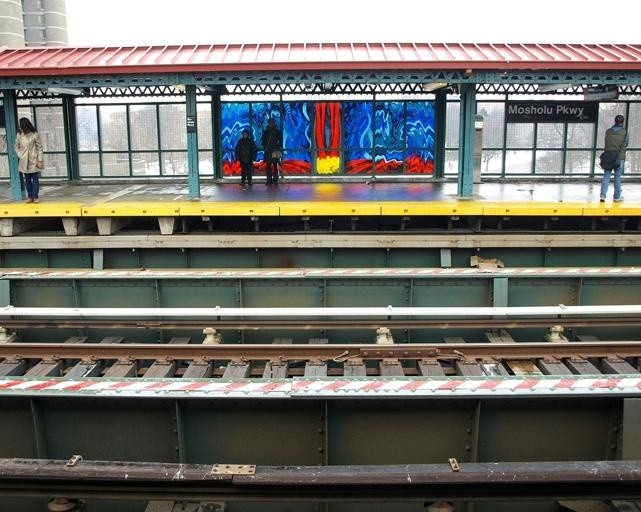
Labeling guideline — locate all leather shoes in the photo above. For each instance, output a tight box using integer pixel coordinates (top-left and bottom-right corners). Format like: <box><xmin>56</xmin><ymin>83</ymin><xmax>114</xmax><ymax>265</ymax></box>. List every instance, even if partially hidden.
<box><xmin>25</xmin><ymin>197</ymin><xmax>39</xmax><ymax>204</ymax></box>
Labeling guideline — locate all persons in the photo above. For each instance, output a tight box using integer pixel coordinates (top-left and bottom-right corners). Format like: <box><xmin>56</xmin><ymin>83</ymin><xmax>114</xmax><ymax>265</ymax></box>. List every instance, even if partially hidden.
<box><xmin>599</xmin><ymin>115</ymin><xmax>628</xmax><ymax>203</ymax></box>
<box><xmin>13</xmin><ymin>118</ymin><xmax>44</xmax><ymax>203</ymax></box>
<box><xmin>234</xmin><ymin>119</ymin><xmax>282</xmax><ymax>191</ymax></box>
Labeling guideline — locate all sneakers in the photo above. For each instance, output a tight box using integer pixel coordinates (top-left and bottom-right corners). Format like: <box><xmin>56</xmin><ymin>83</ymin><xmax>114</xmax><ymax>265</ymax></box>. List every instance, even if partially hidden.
<box><xmin>600</xmin><ymin>197</ymin><xmax>605</xmax><ymax>201</ymax></box>
<box><xmin>267</xmin><ymin>180</ymin><xmax>278</xmax><ymax>185</ymax></box>
<box><xmin>614</xmin><ymin>196</ymin><xmax>624</xmax><ymax>201</ymax></box>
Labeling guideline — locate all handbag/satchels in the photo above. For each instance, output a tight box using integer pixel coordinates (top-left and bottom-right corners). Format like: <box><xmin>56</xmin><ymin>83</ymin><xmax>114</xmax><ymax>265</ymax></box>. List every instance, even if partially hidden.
<box><xmin>600</xmin><ymin>150</ymin><xmax>620</xmax><ymax>169</ymax></box>
<box><xmin>271</xmin><ymin>142</ymin><xmax>282</xmax><ymax>158</ymax></box>
